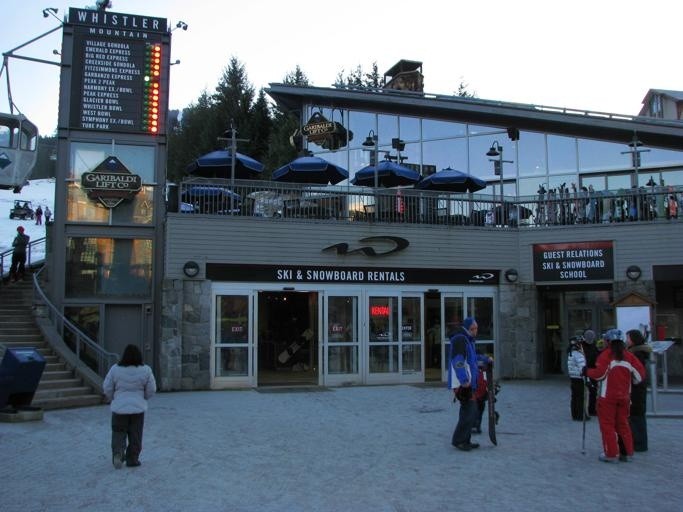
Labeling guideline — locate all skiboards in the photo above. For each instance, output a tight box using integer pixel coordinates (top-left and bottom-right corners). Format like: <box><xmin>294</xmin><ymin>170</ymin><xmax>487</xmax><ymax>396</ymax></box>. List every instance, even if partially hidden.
<box><xmin>535</xmin><ymin>182</ymin><xmax>595</xmax><ymax>225</ymax></box>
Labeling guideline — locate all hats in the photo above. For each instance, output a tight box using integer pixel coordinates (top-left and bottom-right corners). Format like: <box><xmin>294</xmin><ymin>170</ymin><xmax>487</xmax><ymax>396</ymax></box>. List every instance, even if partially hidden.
<box><xmin>585</xmin><ymin>331</ymin><xmax>595</xmax><ymax>344</ymax></box>
<box><xmin>464</xmin><ymin>318</ymin><xmax>474</xmax><ymax>330</ymax></box>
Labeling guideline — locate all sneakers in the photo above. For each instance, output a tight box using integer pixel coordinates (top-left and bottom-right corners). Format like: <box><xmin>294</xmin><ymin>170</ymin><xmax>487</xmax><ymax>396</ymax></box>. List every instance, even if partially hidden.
<box><xmin>127</xmin><ymin>461</ymin><xmax>141</xmax><ymax>466</ymax></box>
<box><xmin>457</xmin><ymin>442</ymin><xmax>479</xmax><ymax>451</ymax></box>
<box><xmin>620</xmin><ymin>456</ymin><xmax>632</xmax><ymax>461</ymax></box>
<box><xmin>600</xmin><ymin>453</ymin><xmax>616</xmax><ymax>462</ymax></box>
<box><xmin>114</xmin><ymin>452</ymin><xmax>122</xmax><ymax>470</ymax></box>
<box><xmin>471</xmin><ymin>428</ymin><xmax>477</xmax><ymax>433</ymax></box>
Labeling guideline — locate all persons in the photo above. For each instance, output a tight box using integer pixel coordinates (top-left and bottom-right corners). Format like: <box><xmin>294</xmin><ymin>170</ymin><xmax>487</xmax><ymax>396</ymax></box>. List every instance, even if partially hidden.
<box><xmin>424</xmin><ymin>317</ymin><xmax>441</xmax><ymax>369</ymax></box>
<box><xmin>470</xmin><ymin>359</ymin><xmax>491</xmax><ymax>434</ymax></box>
<box><xmin>7</xmin><ymin>226</ymin><xmax>29</xmax><ymax>283</ymax></box>
<box><xmin>22</xmin><ymin>203</ymin><xmax>29</xmax><ymax>209</ymax></box>
<box><xmin>101</xmin><ymin>343</ymin><xmax>157</xmax><ymax>470</ymax></box>
<box><xmin>563</xmin><ymin>326</ymin><xmax>652</xmax><ymax>465</ymax></box>
<box><xmin>215</xmin><ymin>296</ymin><xmax>419</xmax><ymax>379</ymax></box>
<box><xmin>34</xmin><ymin>205</ymin><xmax>42</xmax><ymax>225</ymax></box>
<box><xmin>14</xmin><ymin>202</ymin><xmax>20</xmax><ymax>209</ymax></box>
<box><xmin>446</xmin><ymin>316</ymin><xmax>496</xmax><ymax>451</ymax></box>
<box><xmin>43</xmin><ymin>206</ymin><xmax>51</xmax><ymax>221</ymax></box>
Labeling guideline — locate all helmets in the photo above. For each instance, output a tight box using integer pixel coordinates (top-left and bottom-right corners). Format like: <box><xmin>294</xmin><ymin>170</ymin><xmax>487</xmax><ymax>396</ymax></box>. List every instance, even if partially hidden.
<box><xmin>569</xmin><ymin>336</ymin><xmax>586</xmax><ymax>345</ymax></box>
<box><xmin>597</xmin><ymin>340</ymin><xmax>606</xmax><ymax>348</ymax></box>
<box><xmin>605</xmin><ymin>329</ymin><xmax>625</xmax><ymax>340</ymax></box>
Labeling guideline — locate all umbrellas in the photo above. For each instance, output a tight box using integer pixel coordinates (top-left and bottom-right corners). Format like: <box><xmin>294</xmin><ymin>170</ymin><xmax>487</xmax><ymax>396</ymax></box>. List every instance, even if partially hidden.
<box><xmin>271</xmin><ymin>156</ymin><xmax>349</xmax><ymax>186</ymax></box>
<box><xmin>415</xmin><ymin>166</ymin><xmax>486</xmax><ymax>214</ymax></box>
<box><xmin>179</xmin><ymin>184</ymin><xmax>241</xmax><ymax>213</ymax></box>
<box><xmin>348</xmin><ymin>159</ymin><xmax>421</xmax><ymax>191</ymax></box>
<box><xmin>183</xmin><ymin>147</ymin><xmax>265</xmax><ymax>186</ymax></box>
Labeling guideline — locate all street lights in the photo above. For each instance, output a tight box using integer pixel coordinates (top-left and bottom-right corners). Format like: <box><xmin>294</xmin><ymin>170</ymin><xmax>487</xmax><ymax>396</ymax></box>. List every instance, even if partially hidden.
<box><xmin>622</xmin><ymin>127</ymin><xmax>652</xmax><ymax>222</ymax></box>
<box><xmin>645</xmin><ymin>176</ymin><xmax>657</xmax><ymax>186</ymax></box>
<box><xmin>486</xmin><ymin>140</ymin><xmax>513</xmax><ymax>229</ymax></box>
<box><xmin>359</xmin><ymin>128</ymin><xmax>392</xmax><ymax>225</ymax></box>
<box><xmin>217</xmin><ymin>118</ymin><xmax>250</xmax><ymax>217</ymax></box>
<box><xmin>384</xmin><ymin>136</ymin><xmax>408</xmax><ymax>163</ymax></box>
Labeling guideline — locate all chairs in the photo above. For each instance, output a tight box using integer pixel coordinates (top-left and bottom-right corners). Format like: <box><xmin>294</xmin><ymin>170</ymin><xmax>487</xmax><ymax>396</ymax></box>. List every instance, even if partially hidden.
<box><xmin>241</xmin><ymin>198</ymin><xmax>485</xmax><ymax>226</ymax></box>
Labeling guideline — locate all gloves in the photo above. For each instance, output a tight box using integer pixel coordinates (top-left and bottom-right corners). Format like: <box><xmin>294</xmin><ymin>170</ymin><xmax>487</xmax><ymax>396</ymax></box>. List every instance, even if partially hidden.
<box><xmin>582</xmin><ymin>366</ymin><xmax>588</xmax><ymax>376</ymax></box>
<box><xmin>460</xmin><ymin>386</ymin><xmax>472</xmax><ymax>400</ymax></box>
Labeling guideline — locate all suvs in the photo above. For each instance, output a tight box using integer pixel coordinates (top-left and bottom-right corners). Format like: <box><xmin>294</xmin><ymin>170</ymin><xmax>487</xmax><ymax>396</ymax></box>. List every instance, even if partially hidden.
<box><xmin>9</xmin><ymin>200</ymin><xmax>36</xmax><ymax>221</ymax></box>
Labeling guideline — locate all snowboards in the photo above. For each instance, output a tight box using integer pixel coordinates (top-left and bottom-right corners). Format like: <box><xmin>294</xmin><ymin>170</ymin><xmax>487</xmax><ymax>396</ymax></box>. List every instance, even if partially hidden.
<box><xmin>278</xmin><ymin>328</ymin><xmax>314</xmax><ymax>364</ymax></box>
<box><xmin>486</xmin><ymin>355</ymin><xmax>500</xmax><ymax>446</ymax></box>
